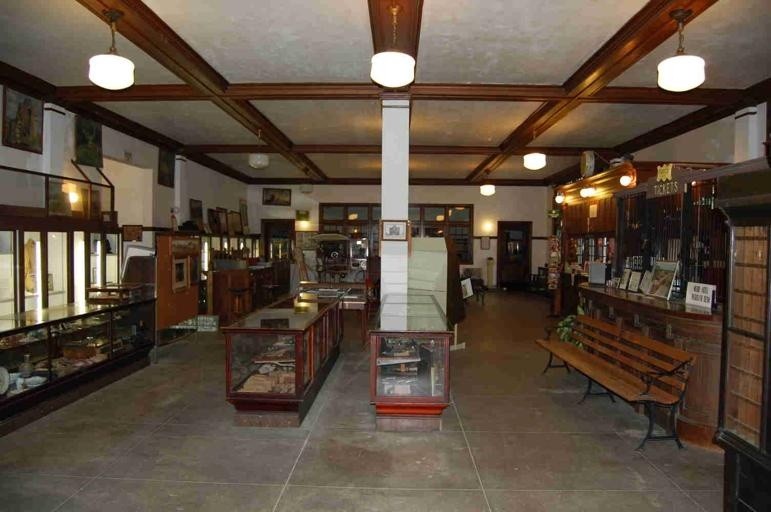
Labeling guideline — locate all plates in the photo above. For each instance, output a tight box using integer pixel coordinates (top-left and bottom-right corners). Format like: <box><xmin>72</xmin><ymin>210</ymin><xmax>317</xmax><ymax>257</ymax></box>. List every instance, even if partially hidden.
<box><xmin>24</xmin><ymin>375</ymin><xmax>48</xmax><ymax>388</ymax></box>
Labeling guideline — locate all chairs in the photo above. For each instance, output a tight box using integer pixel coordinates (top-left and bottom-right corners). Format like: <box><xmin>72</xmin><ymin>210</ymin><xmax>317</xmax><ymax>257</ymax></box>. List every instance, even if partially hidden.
<box><xmin>526</xmin><ymin>266</ymin><xmax>549</xmax><ymax>296</ymax></box>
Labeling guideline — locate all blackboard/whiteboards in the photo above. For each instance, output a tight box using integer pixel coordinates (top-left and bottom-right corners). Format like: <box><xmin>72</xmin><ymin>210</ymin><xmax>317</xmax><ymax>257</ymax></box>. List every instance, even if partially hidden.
<box><xmin>121</xmin><ymin>245</ymin><xmax>156</xmax><ymax>278</ymax></box>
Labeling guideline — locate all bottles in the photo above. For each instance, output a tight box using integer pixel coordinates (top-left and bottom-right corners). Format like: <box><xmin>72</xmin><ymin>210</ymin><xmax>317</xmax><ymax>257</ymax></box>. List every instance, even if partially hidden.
<box><xmin>18</xmin><ymin>354</ymin><xmax>34</xmax><ymax>377</ymax></box>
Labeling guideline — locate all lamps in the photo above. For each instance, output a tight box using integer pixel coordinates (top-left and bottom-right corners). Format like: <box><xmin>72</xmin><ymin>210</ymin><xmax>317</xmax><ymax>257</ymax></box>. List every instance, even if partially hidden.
<box><xmin>522</xmin><ymin>130</ymin><xmax>548</xmax><ymax>170</ymax></box>
<box><xmin>88</xmin><ymin>7</ymin><xmax>135</xmax><ymax>91</ymax></box>
<box><xmin>658</xmin><ymin>10</ymin><xmax>707</xmax><ymax>92</ymax></box>
<box><xmin>368</xmin><ymin>5</ymin><xmax>416</xmax><ymax>87</ymax></box>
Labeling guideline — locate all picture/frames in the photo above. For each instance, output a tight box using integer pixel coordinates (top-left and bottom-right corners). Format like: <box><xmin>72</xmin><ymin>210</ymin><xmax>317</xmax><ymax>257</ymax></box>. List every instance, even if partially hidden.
<box><xmin>262</xmin><ymin>187</ymin><xmax>293</xmax><ymax>206</ymax></box>
<box><xmin>380</xmin><ymin>220</ymin><xmax>410</xmax><ymax>242</ymax></box>
<box><xmin>1</xmin><ymin>85</ymin><xmax>44</xmax><ymax>153</ymax></box>
<box><xmin>618</xmin><ymin>259</ymin><xmax>681</xmax><ymax>301</ymax></box>
<box><xmin>189</xmin><ymin>198</ymin><xmax>243</xmax><ymax>235</ymax></box>
<box><xmin>171</xmin><ymin>255</ymin><xmax>190</xmax><ymax>294</ymax></box>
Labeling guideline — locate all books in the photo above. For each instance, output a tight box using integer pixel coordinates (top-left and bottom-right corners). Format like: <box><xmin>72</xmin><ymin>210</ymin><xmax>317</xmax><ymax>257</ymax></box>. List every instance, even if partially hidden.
<box><xmin>240</xmin><ymin>337</ymin><xmax>295</xmax><ymax>394</ymax></box>
<box><xmin>377</xmin><ymin>346</ymin><xmax>422</xmax><ymax>395</ymax></box>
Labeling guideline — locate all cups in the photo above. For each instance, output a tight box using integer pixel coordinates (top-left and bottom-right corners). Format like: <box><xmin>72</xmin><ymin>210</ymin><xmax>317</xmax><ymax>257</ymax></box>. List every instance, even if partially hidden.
<box><xmin>16</xmin><ymin>378</ymin><xmax>24</xmax><ymax>392</ymax></box>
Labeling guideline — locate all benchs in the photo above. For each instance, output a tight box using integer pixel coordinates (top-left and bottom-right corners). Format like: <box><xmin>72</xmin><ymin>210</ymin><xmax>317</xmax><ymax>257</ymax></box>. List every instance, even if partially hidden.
<box><xmin>535</xmin><ymin>310</ymin><xmax>700</xmax><ymax>455</ymax></box>
